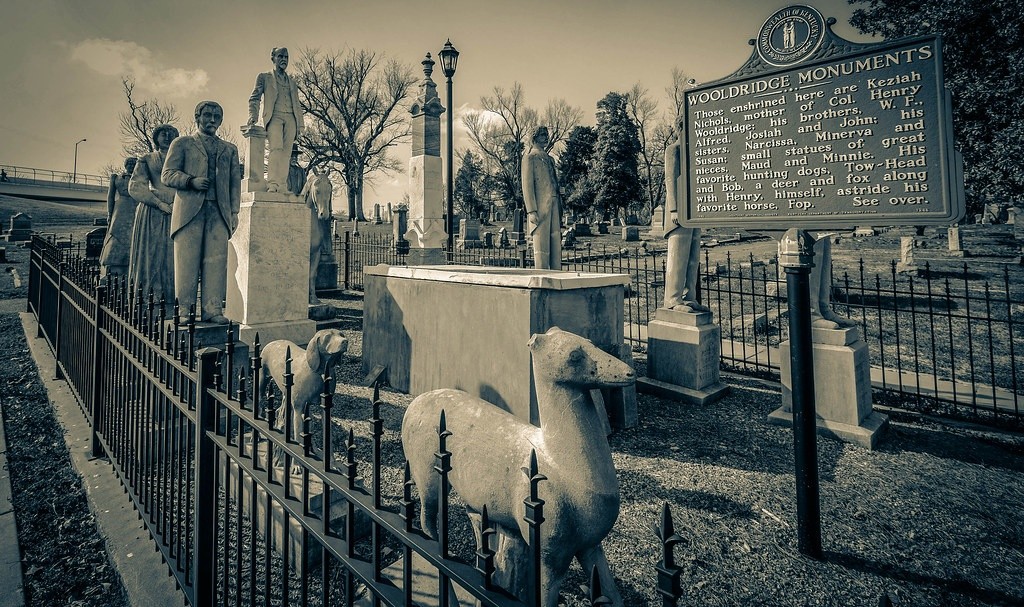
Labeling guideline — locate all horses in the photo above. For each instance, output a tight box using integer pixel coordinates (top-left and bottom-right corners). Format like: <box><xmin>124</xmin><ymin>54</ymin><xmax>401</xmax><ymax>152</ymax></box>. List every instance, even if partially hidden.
<box><xmin>298</xmin><ymin>167</ymin><xmax>333</xmax><ymax>302</ymax></box>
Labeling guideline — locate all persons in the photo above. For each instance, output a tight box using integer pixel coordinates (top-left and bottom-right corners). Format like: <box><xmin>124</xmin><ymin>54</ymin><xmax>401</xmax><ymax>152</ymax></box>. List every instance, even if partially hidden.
<box><xmin>664</xmin><ymin>117</ymin><xmax>858</xmax><ymax>329</ymax></box>
<box><xmin>520</xmin><ymin>127</ymin><xmax>561</xmax><ymax>269</ymax></box>
<box><xmin>104</xmin><ymin>47</ymin><xmax>311</xmax><ymax>325</ymax></box>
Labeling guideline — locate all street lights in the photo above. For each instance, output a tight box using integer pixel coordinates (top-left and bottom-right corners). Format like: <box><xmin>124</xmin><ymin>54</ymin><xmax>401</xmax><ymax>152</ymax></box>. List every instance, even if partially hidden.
<box><xmin>437</xmin><ymin>37</ymin><xmax>461</xmax><ymax>266</ymax></box>
<box><xmin>73</xmin><ymin>139</ymin><xmax>88</xmax><ymax>183</ymax></box>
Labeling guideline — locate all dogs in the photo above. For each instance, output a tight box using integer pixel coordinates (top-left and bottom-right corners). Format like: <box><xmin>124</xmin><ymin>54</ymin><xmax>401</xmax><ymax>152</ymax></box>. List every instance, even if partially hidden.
<box><xmin>259</xmin><ymin>328</ymin><xmax>348</xmax><ymax>442</ymax></box>
